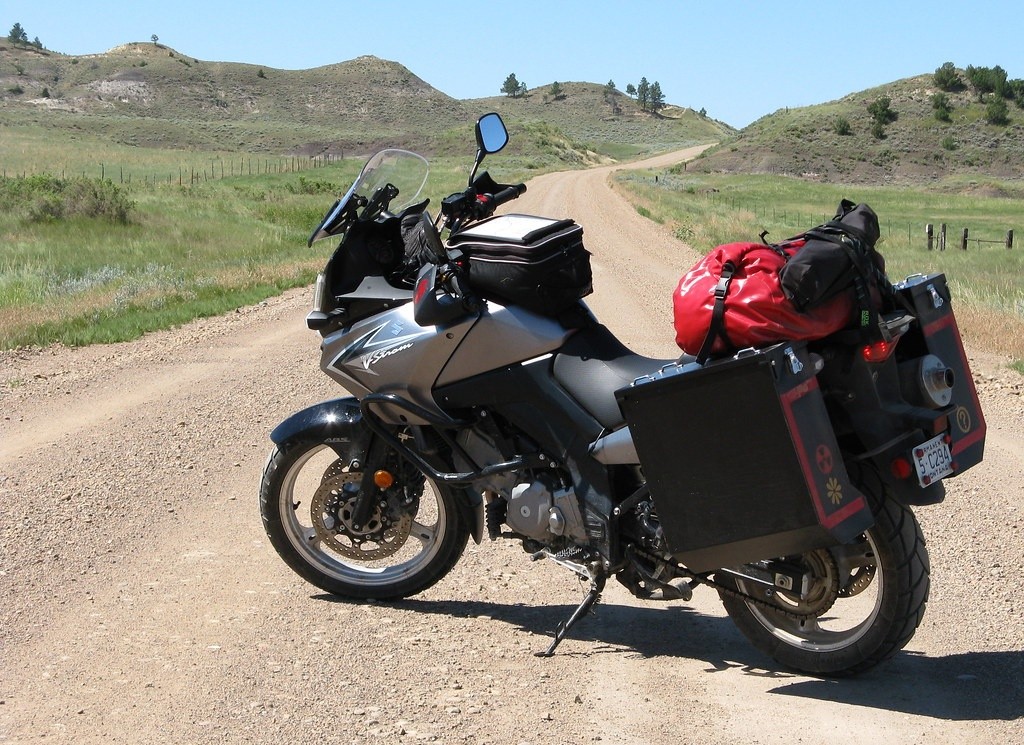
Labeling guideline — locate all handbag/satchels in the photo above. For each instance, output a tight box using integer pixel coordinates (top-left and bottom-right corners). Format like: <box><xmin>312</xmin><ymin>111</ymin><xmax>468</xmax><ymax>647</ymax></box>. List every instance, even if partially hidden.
<box><xmin>672</xmin><ymin>198</ymin><xmax>895</xmax><ymax>358</ymax></box>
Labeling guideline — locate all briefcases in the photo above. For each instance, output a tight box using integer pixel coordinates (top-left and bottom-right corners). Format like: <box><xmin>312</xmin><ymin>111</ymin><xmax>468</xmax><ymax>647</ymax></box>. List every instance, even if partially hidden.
<box><xmin>443</xmin><ymin>214</ymin><xmax>593</xmax><ymax>311</ymax></box>
<box><xmin>800</xmin><ymin>272</ymin><xmax>987</xmax><ymax>477</ymax></box>
<box><xmin>613</xmin><ymin>342</ymin><xmax>874</xmax><ymax>574</ymax></box>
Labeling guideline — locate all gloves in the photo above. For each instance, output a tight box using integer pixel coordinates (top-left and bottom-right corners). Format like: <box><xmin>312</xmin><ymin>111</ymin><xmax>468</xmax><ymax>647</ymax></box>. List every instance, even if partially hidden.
<box><xmin>399</xmin><ymin>213</ymin><xmax>445</xmax><ymax>268</ymax></box>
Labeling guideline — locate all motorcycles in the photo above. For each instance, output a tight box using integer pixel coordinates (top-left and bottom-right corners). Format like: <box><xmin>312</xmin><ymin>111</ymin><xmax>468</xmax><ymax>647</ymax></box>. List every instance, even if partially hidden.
<box><xmin>259</xmin><ymin>111</ymin><xmax>988</xmax><ymax>681</ymax></box>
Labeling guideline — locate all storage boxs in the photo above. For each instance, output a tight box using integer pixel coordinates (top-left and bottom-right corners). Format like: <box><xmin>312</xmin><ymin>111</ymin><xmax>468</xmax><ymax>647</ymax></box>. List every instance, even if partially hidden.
<box><xmin>887</xmin><ymin>275</ymin><xmax>987</xmax><ymax>478</ymax></box>
<box><xmin>613</xmin><ymin>340</ymin><xmax>874</xmax><ymax>571</ymax></box>
